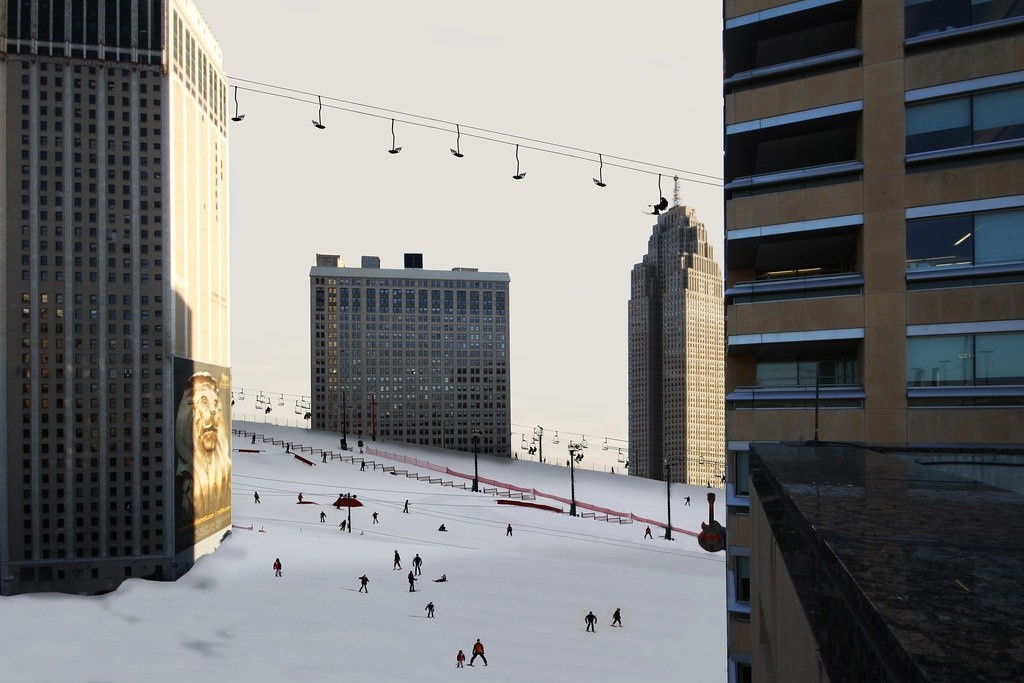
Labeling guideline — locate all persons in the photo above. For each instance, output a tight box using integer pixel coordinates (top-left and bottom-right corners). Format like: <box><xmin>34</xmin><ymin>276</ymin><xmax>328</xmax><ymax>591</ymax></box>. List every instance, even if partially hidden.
<box><xmin>570</xmin><ymin>443</ymin><xmax>583</xmax><ymax>461</ymax></box>
<box><xmin>612</xmin><ymin>608</ymin><xmax>621</xmax><ymax>626</ymax></box>
<box><xmin>566</xmin><ymin>460</ymin><xmax>570</xmax><ymax>467</ymax></box>
<box><xmin>322</xmin><ymin>452</ymin><xmax>326</xmax><ymax>463</ymax></box>
<box><xmin>625</xmin><ymin>461</ymin><xmax>629</xmax><ymax>468</ymax></box>
<box><xmin>469</xmin><ymin>639</ymin><xmax>487</xmax><ymax>666</ymax></box>
<box><xmin>254</xmin><ymin>490</ymin><xmax>512</xmax><ymax>619</ymax></box>
<box><xmin>266</xmin><ymin>407</ymin><xmax>270</xmax><ymax>413</ymax></box>
<box><xmin>457</xmin><ymin>650</ymin><xmax>465</xmax><ymax>668</ymax></box>
<box><xmin>721</xmin><ymin>475</ymin><xmax>726</xmax><ymax>483</ymax></box>
<box><xmin>286</xmin><ymin>443</ymin><xmax>289</xmax><ymax>453</ymax></box>
<box><xmin>611</xmin><ymin>467</ymin><xmax>615</xmax><ymax>474</ymax></box>
<box><xmin>651</xmin><ymin>197</ymin><xmax>668</xmax><ymax>215</ymax></box>
<box><xmin>304</xmin><ymin>412</ymin><xmax>311</xmax><ymax>419</ymax></box>
<box><xmin>252</xmin><ymin>433</ymin><xmax>256</xmax><ymax>444</ymax></box>
<box><xmin>360</xmin><ymin>461</ymin><xmax>365</xmax><ymax>471</ymax></box>
<box><xmin>644</xmin><ymin>525</ymin><xmax>652</xmax><ymax>539</ymax></box>
<box><xmin>585</xmin><ymin>611</ymin><xmax>597</xmax><ymax>632</ymax></box>
<box><xmin>684</xmin><ymin>496</ymin><xmax>690</xmax><ymax>506</ymax></box>
<box><xmin>514</xmin><ymin>452</ymin><xmax>518</xmax><ymax>460</ymax></box>
<box><xmin>528</xmin><ymin>446</ymin><xmax>536</xmax><ymax>454</ymax></box>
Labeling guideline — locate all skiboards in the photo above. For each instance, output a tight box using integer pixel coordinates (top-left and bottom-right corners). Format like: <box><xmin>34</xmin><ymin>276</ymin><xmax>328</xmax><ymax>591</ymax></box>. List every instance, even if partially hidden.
<box><xmin>611</xmin><ymin>625</ymin><xmax>623</xmax><ymax>627</ymax></box>
<box><xmin>585</xmin><ymin>630</ymin><xmax>595</xmax><ymax>633</ymax></box>
<box><xmin>457</xmin><ymin>666</ymin><xmax>463</xmax><ymax>668</ymax></box>
<box><xmin>393</xmin><ymin>568</ymin><xmax>403</xmax><ymax>571</ymax></box>
<box><xmin>414</xmin><ymin>574</ymin><xmax>422</xmax><ymax>576</ymax></box>
<box><xmin>468</xmin><ymin>664</ymin><xmax>488</xmax><ymax>667</ymax></box>
